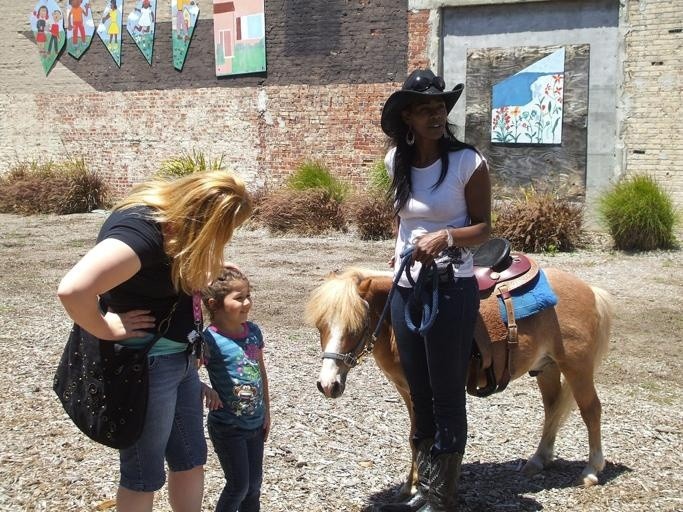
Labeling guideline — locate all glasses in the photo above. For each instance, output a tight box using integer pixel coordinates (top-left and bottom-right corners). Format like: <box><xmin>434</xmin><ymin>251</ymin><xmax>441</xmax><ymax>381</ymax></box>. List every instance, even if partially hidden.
<box><xmin>409</xmin><ymin>76</ymin><xmax>445</xmax><ymax>92</ymax></box>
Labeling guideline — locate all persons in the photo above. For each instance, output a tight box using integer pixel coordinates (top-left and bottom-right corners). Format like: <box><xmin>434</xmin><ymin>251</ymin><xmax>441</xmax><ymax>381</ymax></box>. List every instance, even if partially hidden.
<box><xmin>196</xmin><ymin>261</ymin><xmax>271</xmax><ymax>512</ymax></box>
<box><xmin>55</xmin><ymin>169</ymin><xmax>255</xmax><ymax>512</ymax></box>
<box><xmin>380</xmin><ymin>68</ymin><xmax>494</xmax><ymax>512</ymax></box>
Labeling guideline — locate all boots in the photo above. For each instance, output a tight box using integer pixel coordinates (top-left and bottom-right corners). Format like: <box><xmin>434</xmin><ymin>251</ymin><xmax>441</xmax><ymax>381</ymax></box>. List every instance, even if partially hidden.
<box><xmin>378</xmin><ymin>439</ymin><xmax>463</xmax><ymax>512</ymax></box>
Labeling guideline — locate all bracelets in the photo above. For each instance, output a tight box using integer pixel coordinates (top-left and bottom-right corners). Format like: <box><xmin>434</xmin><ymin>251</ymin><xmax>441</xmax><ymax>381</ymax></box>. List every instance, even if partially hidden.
<box><xmin>444</xmin><ymin>228</ymin><xmax>454</xmax><ymax>247</ymax></box>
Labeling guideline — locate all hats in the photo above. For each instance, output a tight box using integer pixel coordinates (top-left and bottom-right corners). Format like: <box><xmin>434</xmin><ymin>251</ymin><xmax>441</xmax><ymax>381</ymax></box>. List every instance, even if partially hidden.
<box><xmin>381</xmin><ymin>70</ymin><xmax>464</xmax><ymax>138</ymax></box>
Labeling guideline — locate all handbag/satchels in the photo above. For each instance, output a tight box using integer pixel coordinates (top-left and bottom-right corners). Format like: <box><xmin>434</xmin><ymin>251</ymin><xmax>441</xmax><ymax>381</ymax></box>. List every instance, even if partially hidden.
<box><xmin>53</xmin><ymin>323</ymin><xmax>162</xmax><ymax>448</ymax></box>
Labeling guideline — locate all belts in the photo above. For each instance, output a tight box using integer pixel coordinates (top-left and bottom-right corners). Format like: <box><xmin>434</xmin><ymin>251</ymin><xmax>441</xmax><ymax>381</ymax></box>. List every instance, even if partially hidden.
<box><xmin>190</xmin><ymin>344</ymin><xmax>198</xmax><ymax>355</ymax></box>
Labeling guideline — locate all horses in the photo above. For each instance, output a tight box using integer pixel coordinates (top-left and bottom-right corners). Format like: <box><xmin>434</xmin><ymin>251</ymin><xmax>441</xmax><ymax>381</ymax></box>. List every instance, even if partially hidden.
<box><xmin>301</xmin><ymin>265</ymin><xmax>615</xmax><ymax>503</ymax></box>
